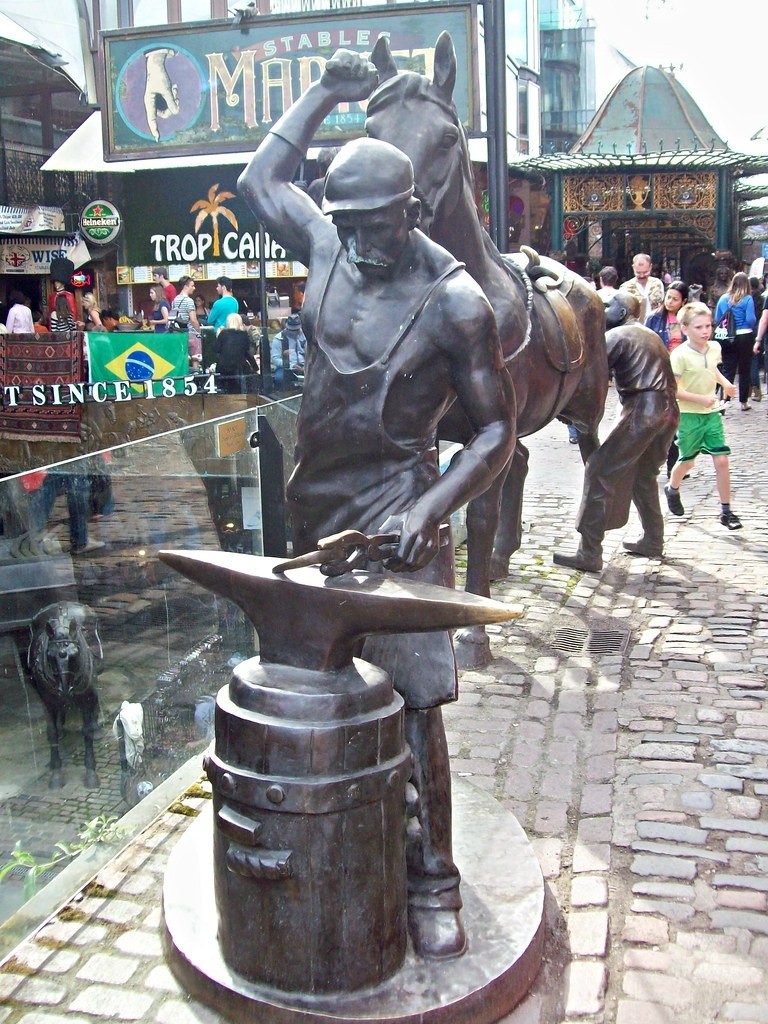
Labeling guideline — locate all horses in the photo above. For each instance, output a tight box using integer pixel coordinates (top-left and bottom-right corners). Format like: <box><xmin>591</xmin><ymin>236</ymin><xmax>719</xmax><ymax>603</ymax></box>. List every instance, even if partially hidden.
<box><xmin>363</xmin><ymin>31</ymin><xmax>609</xmax><ymax>677</ymax></box>
<box><xmin>25</xmin><ymin>599</ymin><xmax>109</xmax><ymax>792</ymax></box>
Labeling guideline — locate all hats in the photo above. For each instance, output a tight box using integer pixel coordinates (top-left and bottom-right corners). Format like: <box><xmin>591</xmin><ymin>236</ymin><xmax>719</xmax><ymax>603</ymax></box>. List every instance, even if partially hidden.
<box><xmin>50</xmin><ymin>258</ymin><xmax>74</xmax><ymax>285</ymax></box>
<box><xmin>152</xmin><ymin>268</ymin><xmax>167</xmax><ymax>274</ymax></box>
<box><xmin>286</xmin><ymin>313</ymin><xmax>302</xmax><ymax>330</ymax></box>
<box><xmin>750</xmin><ymin>277</ymin><xmax>764</xmax><ymax>298</ymax></box>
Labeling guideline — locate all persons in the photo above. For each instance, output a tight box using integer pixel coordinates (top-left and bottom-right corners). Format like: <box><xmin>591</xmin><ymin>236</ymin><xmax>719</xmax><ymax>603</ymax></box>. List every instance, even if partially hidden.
<box><xmin>26</xmin><ymin>457</ymin><xmax>114</xmax><ymax>555</ymax></box>
<box><xmin>149</xmin><ymin>285</ymin><xmax>171</xmax><ymax>333</ymax></box>
<box><xmin>270</xmin><ymin>314</ymin><xmax>307</xmax><ymax>391</ymax></box>
<box><xmin>664</xmin><ymin>301</ymin><xmax>743</xmax><ymax>530</ymax></box>
<box><xmin>167</xmin><ymin>276</ymin><xmax>203</xmax><ymax>368</ymax></box>
<box><xmin>81</xmin><ymin>291</ymin><xmax>109</xmax><ymax>333</ymax></box>
<box><xmin>714</xmin><ymin>271</ymin><xmax>757</xmax><ymax>415</ymax></box>
<box><xmin>213</xmin><ymin>312</ymin><xmax>259</xmax><ymax>394</ymax></box>
<box><xmin>152</xmin><ymin>267</ymin><xmax>177</xmax><ymax>307</ymax></box>
<box><xmin>0</xmin><ymin>291</ymin><xmax>49</xmax><ymax>335</ymax></box>
<box><xmin>553</xmin><ymin>291</ymin><xmax>681</xmax><ymax>573</ymax></box>
<box><xmin>618</xmin><ymin>252</ymin><xmax>665</xmax><ymax>328</ymax></box>
<box><xmin>194</xmin><ymin>294</ymin><xmax>211</xmax><ymax>326</ymax></box>
<box><xmin>643</xmin><ymin>281</ymin><xmax>689</xmax><ymax>479</ymax></box>
<box><xmin>236</xmin><ymin>46</ymin><xmax>516</xmax><ymax>959</ymax></box>
<box><xmin>46</xmin><ymin>279</ymin><xmax>76</xmax><ymax>330</ymax></box>
<box><xmin>567</xmin><ymin>250</ymin><xmax>768</xmax><ymax>479</ymax></box>
<box><xmin>207</xmin><ymin>276</ymin><xmax>239</xmax><ymax>337</ymax></box>
<box><xmin>50</xmin><ymin>294</ymin><xmax>76</xmax><ymax>333</ymax></box>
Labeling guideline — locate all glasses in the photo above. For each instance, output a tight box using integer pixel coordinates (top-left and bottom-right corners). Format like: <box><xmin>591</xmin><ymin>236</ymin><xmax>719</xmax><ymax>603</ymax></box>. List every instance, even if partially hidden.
<box><xmin>634</xmin><ymin>267</ymin><xmax>651</xmax><ymax>277</ymax></box>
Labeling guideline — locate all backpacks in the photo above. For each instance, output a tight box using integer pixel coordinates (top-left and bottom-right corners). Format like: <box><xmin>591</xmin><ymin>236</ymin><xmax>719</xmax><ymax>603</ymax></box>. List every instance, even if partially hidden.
<box><xmin>711</xmin><ymin>295</ymin><xmax>736</xmax><ymax>349</ymax></box>
<box><xmin>165</xmin><ymin>297</ymin><xmax>190</xmax><ymax>328</ymax></box>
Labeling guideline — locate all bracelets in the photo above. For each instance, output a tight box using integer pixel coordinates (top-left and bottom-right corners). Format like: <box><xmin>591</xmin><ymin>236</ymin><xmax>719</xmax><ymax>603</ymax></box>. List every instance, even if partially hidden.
<box><xmin>755</xmin><ymin>337</ymin><xmax>762</xmax><ymax>341</ymax></box>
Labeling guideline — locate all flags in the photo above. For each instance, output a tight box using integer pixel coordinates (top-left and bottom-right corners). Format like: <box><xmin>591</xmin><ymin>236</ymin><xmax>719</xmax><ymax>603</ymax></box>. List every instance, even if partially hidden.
<box><xmin>86</xmin><ymin>331</ymin><xmax>189</xmax><ymax>395</ymax></box>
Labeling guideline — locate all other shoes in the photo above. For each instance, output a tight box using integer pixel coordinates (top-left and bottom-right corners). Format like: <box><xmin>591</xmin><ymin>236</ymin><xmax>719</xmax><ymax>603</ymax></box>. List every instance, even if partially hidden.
<box><xmin>664</xmin><ymin>483</ymin><xmax>684</xmax><ymax>516</ymax></box>
<box><xmin>741</xmin><ymin>403</ymin><xmax>751</xmax><ymax>411</ymax></box>
<box><xmin>71</xmin><ymin>537</ymin><xmax>105</xmax><ymax>554</ymax></box>
<box><xmin>569</xmin><ymin>435</ymin><xmax>578</xmax><ymax>444</ymax></box>
<box><xmin>667</xmin><ymin>470</ymin><xmax>690</xmax><ymax>480</ymax></box>
<box><xmin>751</xmin><ymin>389</ymin><xmax>763</xmax><ymax>401</ymax></box>
<box><xmin>720</xmin><ymin>513</ymin><xmax>742</xmax><ymax>530</ymax></box>
<box><xmin>720</xmin><ymin>409</ymin><xmax>725</xmax><ymax>416</ymax></box>
